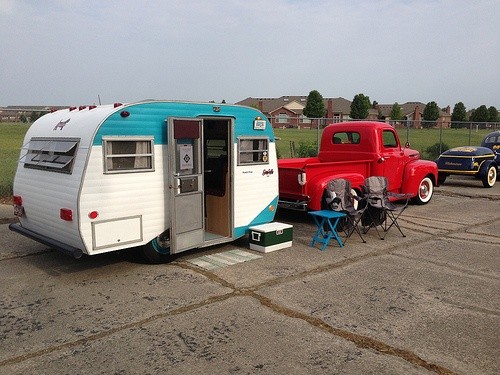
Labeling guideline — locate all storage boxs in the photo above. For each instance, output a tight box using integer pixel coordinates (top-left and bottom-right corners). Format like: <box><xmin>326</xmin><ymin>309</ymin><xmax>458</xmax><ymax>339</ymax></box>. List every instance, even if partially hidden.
<box><xmin>249</xmin><ymin>222</ymin><xmax>294</xmax><ymax>253</ymax></box>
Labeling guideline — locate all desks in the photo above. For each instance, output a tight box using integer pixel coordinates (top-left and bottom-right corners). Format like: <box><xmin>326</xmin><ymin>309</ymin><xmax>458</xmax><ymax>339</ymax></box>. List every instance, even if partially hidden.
<box><xmin>307</xmin><ymin>210</ymin><xmax>347</xmax><ymax>250</ymax></box>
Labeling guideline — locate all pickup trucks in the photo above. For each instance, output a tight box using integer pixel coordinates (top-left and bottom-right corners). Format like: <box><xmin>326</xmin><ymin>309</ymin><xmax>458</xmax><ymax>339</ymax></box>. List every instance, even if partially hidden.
<box><xmin>278</xmin><ymin>120</ymin><xmax>440</xmax><ymax>229</ymax></box>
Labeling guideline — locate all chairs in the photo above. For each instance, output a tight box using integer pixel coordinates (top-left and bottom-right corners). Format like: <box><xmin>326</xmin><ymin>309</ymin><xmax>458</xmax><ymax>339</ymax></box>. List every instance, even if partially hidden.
<box><xmin>362</xmin><ymin>177</ymin><xmax>415</xmax><ymax>240</ymax></box>
<box><xmin>325</xmin><ymin>176</ymin><xmax>370</xmax><ymax>243</ymax></box>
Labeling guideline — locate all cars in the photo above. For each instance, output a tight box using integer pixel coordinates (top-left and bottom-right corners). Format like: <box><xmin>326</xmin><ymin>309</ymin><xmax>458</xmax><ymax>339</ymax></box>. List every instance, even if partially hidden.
<box><xmin>436</xmin><ymin>131</ymin><xmax>500</xmax><ymax>188</ymax></box>
